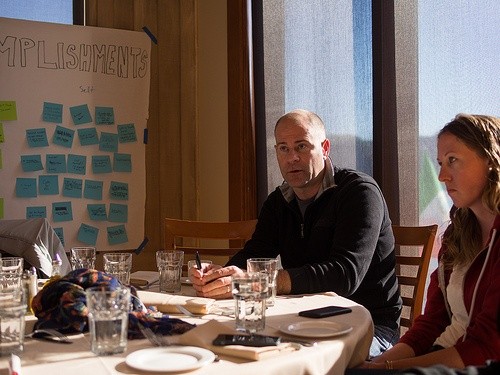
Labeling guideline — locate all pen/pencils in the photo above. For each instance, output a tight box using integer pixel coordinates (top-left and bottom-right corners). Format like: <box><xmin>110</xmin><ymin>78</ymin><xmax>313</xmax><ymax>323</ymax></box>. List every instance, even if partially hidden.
<box><xmin>196</xmin><ymin>250</ymin><xmax>202</xmax><ymax>270</ymax></box>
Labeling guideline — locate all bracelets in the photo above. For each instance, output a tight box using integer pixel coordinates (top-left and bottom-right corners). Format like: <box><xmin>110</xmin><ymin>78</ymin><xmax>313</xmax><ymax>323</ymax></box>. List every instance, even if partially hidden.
<box><xmin>368</xmin><ymin>363</ymin><xmax>372</xmax><ymax>369</ymax></box>
<box><xmin>385</xmin><ymin>360</ymin><xmax>392</xmax><ymax>369</ymax></box>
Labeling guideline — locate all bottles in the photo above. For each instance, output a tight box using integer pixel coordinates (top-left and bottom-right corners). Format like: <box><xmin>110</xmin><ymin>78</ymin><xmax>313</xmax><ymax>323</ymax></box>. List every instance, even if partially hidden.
<box><xmin>22</xmin><ymin>266</ymin><xmax>38</xmax><ymax>315</ymax></box>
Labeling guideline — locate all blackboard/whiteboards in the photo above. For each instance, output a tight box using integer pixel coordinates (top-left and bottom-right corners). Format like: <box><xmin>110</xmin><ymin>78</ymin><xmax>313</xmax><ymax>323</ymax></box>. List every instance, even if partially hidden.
<box><xmin>0</xmin><ymin>20</ymin><xmax>153</xmax><ymax>253</ymax></box>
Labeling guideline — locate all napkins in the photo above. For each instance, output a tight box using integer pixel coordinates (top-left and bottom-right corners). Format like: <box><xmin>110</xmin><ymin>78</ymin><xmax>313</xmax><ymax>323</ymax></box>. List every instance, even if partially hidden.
<box><xmin>179</xmin><ymin>319</ymin><xmax>296</xmax><ymax>360</ymax></box>
<box><xmin>130</xmin><ymin>270</ymin><xmax>160</xmax><ymax>287</ymax></box>
<box><xmin>143</xmin><ymin>294</ymin><xmax>216</xmax><ymax>314</ymax></box>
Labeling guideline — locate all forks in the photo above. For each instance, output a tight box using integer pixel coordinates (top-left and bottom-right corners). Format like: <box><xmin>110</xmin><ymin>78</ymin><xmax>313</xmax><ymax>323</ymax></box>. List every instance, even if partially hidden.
<box><xmin>24</xmin><ymin>328</ymin><xmax>74</xmax><ymax>343</ymax></box>
<box><xmin>136</xmin><ymin>322</ymin><xmax>188</xmax><ymax>345</ymax></box>
<box><xmin>176</xmin><ymin>304</ymin><xmax>214</xmax><ymax>317</ymax></box>
<box><xmin>129</xmin><ymin>279</ymin><xmax>160</xmax><ymax>292</ymax></box>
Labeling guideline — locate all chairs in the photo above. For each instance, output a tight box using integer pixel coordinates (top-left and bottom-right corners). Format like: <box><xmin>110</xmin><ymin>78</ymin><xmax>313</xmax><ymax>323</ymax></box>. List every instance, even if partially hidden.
<box><xmin>392</xmin><ymin>224</ymin><xmax>438</xmax><ymax>330</ymax></box>
<box><xmin>165</xmin><ymin>218</ymin><xmax>258</xmax><ymax>277</ymax></box>
<box><xmin>0</xmin><ymin>218</ymin><xmax>48</xmax><ymax>279</ymax></box>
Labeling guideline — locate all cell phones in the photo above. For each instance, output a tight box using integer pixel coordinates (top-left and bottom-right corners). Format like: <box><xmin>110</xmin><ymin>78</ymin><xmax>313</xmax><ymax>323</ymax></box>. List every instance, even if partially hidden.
<box><xmin>298</xmin><ymin>306</ymin><xmax>352</xmax><ymax>319</ymax></box>
<box><xmin>212</xmin><ymin>334</ymin><xmax>280</xmax><ymax>346</ymax></box>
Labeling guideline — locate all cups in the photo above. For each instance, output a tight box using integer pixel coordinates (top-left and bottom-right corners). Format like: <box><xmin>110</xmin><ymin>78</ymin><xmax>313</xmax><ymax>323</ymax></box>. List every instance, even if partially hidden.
<box><xmin>155</xmin><ymin>249</ymin><xmax>184</xmax><ymax>295</ymax></box>
<box><xmin>0</xmin><ymin>256</ymin><xmax>23</xmax><ymax>302</ymax></box>
<box><xmin>188</xmin><ymin>259</ymin><xmax>213</xmax><ymax>281</ymax></box>
<box><xmin>230</xmin><ymin>272</ymin><xmax>268</xmax><ymax>331</ymax></box>
<box><xmin>1</xmin><ymin>285</ymin><xmax>28</xmax><ymax>357</ymax></box>
<box><xmin>70</xmin><ymin>247</ymin><xmax>97</xmax><ymax>271</ymax></box>
<box><xmin>246</xmin><ymin>258</ymin><xmax>279</xmax><ymax>307</ymax></box>
<box><xmin>102</xmin><ymin>252</ymin><xmax>133</xmax><ymax>288</ymax></box>
<box><xmin>85</xmin><ymin>286</ymin><xmax>130</xmax><ymax>355</ymax></box>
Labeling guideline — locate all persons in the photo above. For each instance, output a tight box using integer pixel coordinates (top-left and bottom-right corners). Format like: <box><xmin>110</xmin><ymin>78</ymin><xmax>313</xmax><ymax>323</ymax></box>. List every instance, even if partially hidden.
<box><xmin>347</xmin><ymin>114</ymin><xmax>500</xmax><ymax>375</ymax></box>
<box><xmin>191</xmin><ymin>108</ymin><xmax>403</xmax><ymax>358</ymax></box>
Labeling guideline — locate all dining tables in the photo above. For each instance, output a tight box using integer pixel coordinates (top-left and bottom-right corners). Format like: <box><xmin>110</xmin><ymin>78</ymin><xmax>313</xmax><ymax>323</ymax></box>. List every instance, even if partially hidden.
<box><xmin>0</xmin><ymin>277</ymin><xmax>374</xmax><ymax>375</ymax></box>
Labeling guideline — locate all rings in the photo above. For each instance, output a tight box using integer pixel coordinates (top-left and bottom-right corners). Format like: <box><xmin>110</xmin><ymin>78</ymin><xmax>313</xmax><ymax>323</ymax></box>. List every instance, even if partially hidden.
<box><xmin>226</xmin><ymin>286</ymin><xmax>229</xmax><ymax>293</ymax></box>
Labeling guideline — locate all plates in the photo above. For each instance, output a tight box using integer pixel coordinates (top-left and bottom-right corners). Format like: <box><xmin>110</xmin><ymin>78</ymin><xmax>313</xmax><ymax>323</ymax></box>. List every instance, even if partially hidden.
<box><xmin>36</xmin><ymin>278</ymin><xmax>52</xmax><ymax>290</ymax></box>
<box><xmin>123</xmin><ymin>345</ymin><xmax>216</xmax><ymax>373</ymax></box>
<box><xmin>278</xmin><ymin>319</ymin><xmax>354</xmax><ymax>338</ymax></box>
